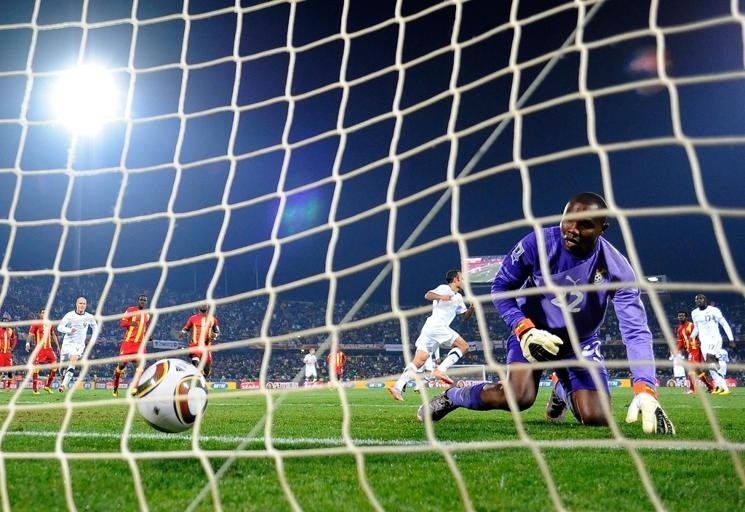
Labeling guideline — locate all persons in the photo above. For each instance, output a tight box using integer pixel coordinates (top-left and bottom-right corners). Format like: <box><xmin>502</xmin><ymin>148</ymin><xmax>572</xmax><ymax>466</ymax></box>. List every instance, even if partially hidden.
<box><xmin>0</xmin><ymin>263</ymin><xmax>745</xmax><ymax>403</ymax></box>
<box><xmin>416</xmin><ymin>192</ymin><xmax>679</xmax><ymax>436</ymax></box>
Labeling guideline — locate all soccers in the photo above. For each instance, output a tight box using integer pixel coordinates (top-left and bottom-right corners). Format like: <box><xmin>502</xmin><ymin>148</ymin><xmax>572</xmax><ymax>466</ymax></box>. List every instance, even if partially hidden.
<box><xmin>135</xmin><ymin>360</ymin><xmax>210</xmax><ymax>431</ymax></box>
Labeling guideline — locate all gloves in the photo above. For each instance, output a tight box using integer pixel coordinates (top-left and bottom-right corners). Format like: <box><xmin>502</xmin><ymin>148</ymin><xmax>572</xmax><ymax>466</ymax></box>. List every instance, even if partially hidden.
<box><xmin>514</xmin><ymin>319</ymin><xmax>564</xmax><ymax>363</ymax></box>
<box><xmin>625</xmin><ymin>384</ymin><xmax>676</xmax><ymax>435</ymax></box>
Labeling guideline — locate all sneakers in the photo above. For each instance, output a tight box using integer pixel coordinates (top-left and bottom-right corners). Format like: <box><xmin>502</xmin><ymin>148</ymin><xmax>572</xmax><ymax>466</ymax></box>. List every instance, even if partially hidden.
<box><xmin>387</xmin><ymin>368</ymin><xmax>454</xmax><ymax>400</ymax></box>
<box><xmin>0</xmin><ymin>375</ymin><xmax>64</xmax><ymax>395</ymax></box>
<box><xmin>687</xmin><ymin>383</ymin><xmax>731</xmax><ymax>395</ymax></box>
<box><xmin>112</xmin><ymin>388</ymin><xmax>137</xmax><ymax>398</ymax></box>
<box><xmin>417</xmin><ymin>386</ymin><xmax>460</xmax><ymax>423</ymax></box>
<box><xmin>547</xmin><ymin>380</ymin><xmax>566</xmax><ymax>419</ymax></box>
